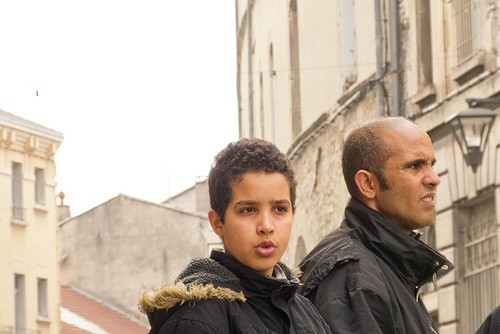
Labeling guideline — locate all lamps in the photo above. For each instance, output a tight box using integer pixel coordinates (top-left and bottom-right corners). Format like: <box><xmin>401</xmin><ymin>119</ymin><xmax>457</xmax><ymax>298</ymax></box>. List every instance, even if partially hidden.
<box><xmin>449</xmin><ymin>96</ymin><xmax>500</xmax><ymax>173</ymax></box>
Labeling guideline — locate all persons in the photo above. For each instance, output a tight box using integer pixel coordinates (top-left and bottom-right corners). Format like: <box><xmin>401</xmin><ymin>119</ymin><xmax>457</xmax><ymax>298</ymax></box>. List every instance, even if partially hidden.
<box><xmin>142</xmin><ymin>134</ymin><xmax>329</xmax><ymax>334</ymax></box>
<box><xmin>292</xmin><ymin>115</ymin><xmax>455</xmax><ymax>334</ymax></box>
